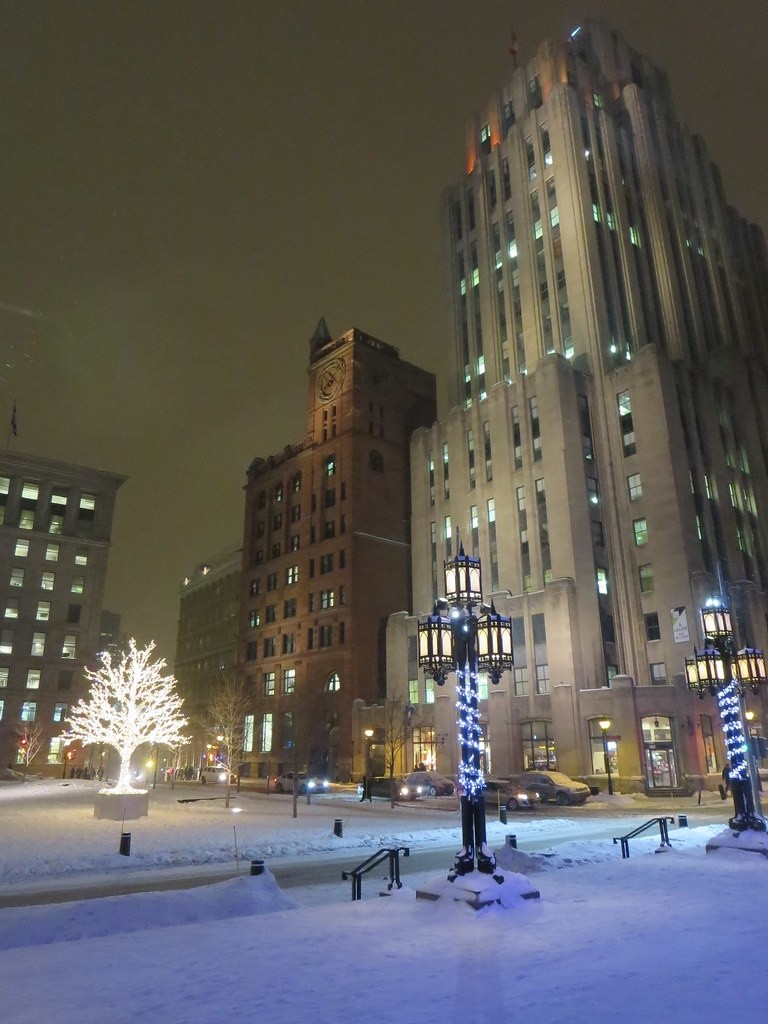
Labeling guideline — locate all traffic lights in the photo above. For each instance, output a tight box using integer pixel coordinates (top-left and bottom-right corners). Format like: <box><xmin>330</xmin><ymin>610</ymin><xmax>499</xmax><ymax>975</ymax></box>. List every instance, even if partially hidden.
<box><xmin>21</xmin><ymin>735</ymin><xmax>27</xmax><ymax>746</ymax></box>
<box><xmin>67</xmin><ymin>749</ymin><xmax>78</xmax><ymax>761</ymax></box>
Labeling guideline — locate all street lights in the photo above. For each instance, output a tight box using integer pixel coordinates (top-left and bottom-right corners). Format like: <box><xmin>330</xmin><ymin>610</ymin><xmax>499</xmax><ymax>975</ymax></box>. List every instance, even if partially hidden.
<box><xmin>600</xmin><ymin>719</ymin><xmax>613</xmax><ymax>795</ymax></box>
<box><xmin>216</xmin><ymin>734</ymin><xmax>225</xmax><ymax>763</ymax></box>
<box><xmin>365</xmin><ymin>729</ymin><xmax>375</xmax><ymax>779</ymax></box>
<box><xmin>678</xmin><ymin>592</ymin><xmax>767</xmax><ymax>853</ymax></box>
<box><xmin>413</xmin><ymin>539</ymin><xmax>541</xmax><ymax>910</ymax></box>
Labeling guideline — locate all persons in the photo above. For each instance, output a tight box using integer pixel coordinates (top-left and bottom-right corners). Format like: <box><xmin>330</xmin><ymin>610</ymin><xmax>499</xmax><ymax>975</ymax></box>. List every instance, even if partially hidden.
<box><xmin>607</xmin><ymin>764</ymin><xmax>613</xmax><ymax>773</ymax></box>
<box><xmin>69</xmin><ymin>766</ymin><xmax>96</xmax><ymax>780</ymax></box>
<box><xmin>721</xmin><ymin>764</ymin><xmax>731</xmax><ymax>793</ymax></box>
<box><xmin>168</xmin><ymin>768</ymin><xmax>175</xmax><ymax>781</ymax></box>
<box><xmin>160</xmin><ymin>768</ymin><xmax>163</xmax><ymax>782</ymax></box>
<box><xmin>414</xmin><ymin>763</ymin><xmax>426</xmax><ymax>772</ymax></box>
<box><xmin>8</xmin><ymin>763</ymin><xmax>13</xmax><ymax>770</ymax></box>
<box><xmin>182</xmin><ymin>766</ymin><xmax>193</xmax><ymax>780</ymax></box>
<box><xmin>360</xmin><ymin>776</ymin><xmax>367</xmax><ymax>803</ymax></box>
<box><xmin>97</xmin><ymin>767</ymin><xmax>104</xmax><ymax>781</ymax></box>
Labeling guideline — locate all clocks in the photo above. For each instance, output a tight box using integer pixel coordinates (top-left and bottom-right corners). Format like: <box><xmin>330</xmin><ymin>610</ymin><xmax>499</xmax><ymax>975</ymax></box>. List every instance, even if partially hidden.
<box><xmin>314</xmin><ymin>357</ymin><xmax>346</xmax><ymax>405</ymax></box>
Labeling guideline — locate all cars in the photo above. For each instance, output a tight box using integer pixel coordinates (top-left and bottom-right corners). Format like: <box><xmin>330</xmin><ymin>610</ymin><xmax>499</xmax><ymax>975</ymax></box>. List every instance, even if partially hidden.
<box><xmin>273</xmin><ymin>772</ymin><xmax>329</xmax><ymax>794</ymax></box>
<box><xmin>483</xmin><ymin>779</ymin><xmax>540</xmax><ymax>809</ymax></box>
<box><xmin>402</xmin><ymin>771</ymin><xmax>455</xmax><ymax>798</ymax></box>
<box><xmin>368</xmin><ymin>776</ymin><xmax>423</xmax><ymax>800</ymax></box>
<box><xmin>502</xmin><ymin>770</ymin><xmax>590</xmax><ymax>805</ymax></box>
<box><xmin>199</xmin><ymin>766</ymin><xmax>237</xmax><ymax>784</ymax></box>
